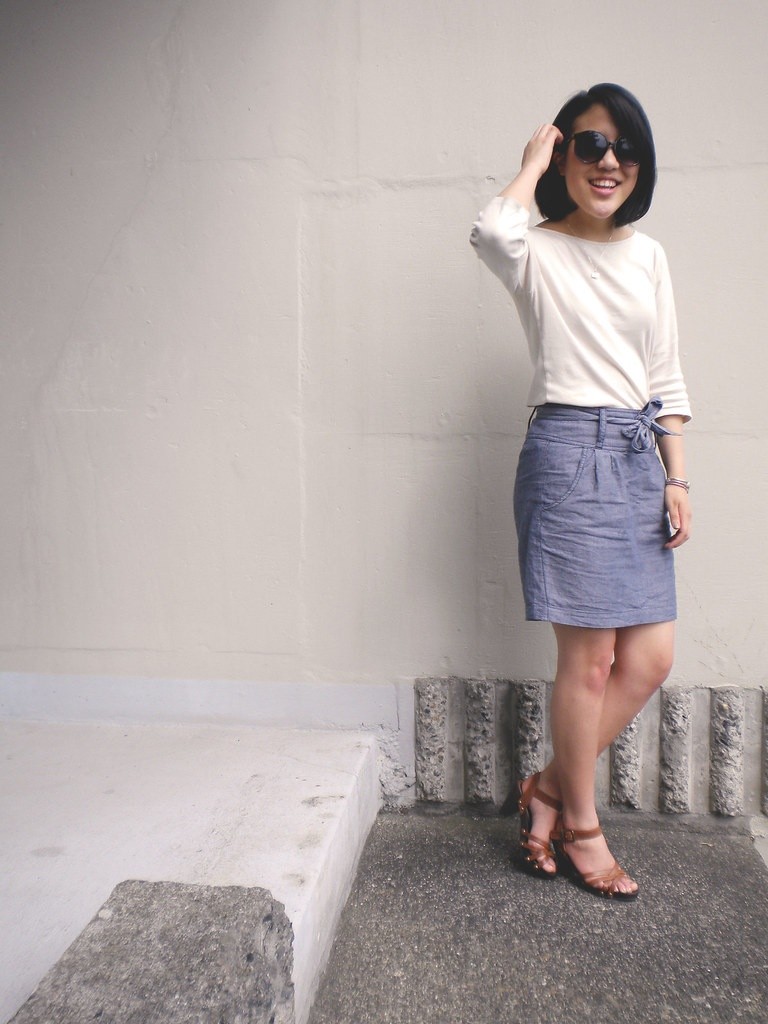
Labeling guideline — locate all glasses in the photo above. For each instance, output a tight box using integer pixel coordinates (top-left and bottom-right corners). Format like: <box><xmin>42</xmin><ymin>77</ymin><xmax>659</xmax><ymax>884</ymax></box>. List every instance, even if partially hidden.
<box><xmin>567</xmin><ymin>130</ymin><xmax>647</xmax><ymax>167</ymax></box>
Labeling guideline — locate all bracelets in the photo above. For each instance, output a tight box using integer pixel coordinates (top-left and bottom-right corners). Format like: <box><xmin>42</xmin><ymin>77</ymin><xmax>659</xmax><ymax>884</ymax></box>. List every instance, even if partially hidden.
<box><xmin>665</xmin><ymin>475</ymin><xmax>691</xmax><ymax>495</ymax></box>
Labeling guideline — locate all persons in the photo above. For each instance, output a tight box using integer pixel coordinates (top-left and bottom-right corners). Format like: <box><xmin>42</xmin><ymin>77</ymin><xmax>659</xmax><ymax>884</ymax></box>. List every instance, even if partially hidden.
<box><xmin>469</xmin><ymin>84</ymin><xmax>694</xmax><ymax>901</ymax></box>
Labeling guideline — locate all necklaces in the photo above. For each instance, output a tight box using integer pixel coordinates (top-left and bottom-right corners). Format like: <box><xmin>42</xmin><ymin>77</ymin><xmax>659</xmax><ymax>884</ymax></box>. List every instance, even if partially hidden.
<box><xmin>566</xmin><ymin>217</ymin><xmax>615</xmax><ymax>279</ymax></box>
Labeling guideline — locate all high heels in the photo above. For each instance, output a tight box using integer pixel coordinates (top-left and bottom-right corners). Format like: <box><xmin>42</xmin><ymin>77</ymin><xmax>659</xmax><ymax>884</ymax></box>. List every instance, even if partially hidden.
<box><xmin>548</xmin><ymin>812</ymin><xmax>642</xmax><ymax>902</ymax></box>
<box><xmin>496</xmin><ymin>768</ymin><xmax>565</xmax><ymax>879</ymax></box>
